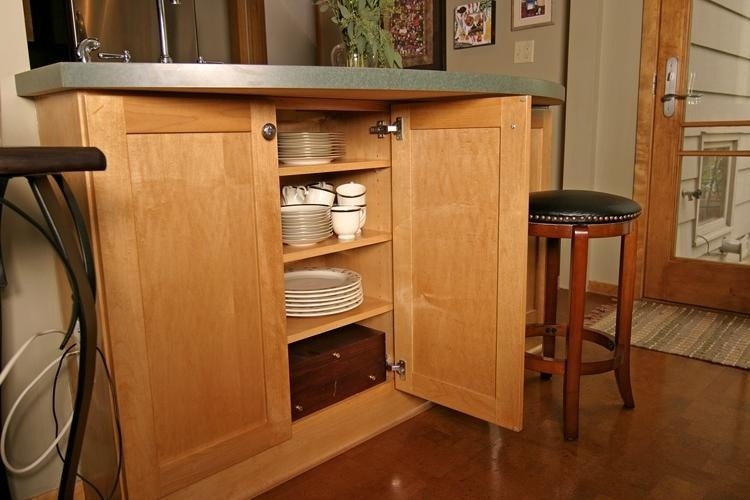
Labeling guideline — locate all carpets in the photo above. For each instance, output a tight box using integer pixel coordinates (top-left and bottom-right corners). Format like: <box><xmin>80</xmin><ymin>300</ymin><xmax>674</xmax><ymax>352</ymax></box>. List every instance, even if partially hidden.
<box><xmin>581</xmin><ymin>297</ymin><xmax>750</xmax><ymax>370</ymax></box>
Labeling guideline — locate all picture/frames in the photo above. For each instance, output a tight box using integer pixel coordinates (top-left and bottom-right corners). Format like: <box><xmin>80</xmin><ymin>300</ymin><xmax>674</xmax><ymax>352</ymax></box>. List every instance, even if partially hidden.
<box><xmin>510</xmin><ymin>0</ymin><xmax>554</xmax><ymax>32</ymax></box>
<box><xmin>378</xmin><ymin>0</ymin><xmax>447</xmax><ymax>71</ymax></box>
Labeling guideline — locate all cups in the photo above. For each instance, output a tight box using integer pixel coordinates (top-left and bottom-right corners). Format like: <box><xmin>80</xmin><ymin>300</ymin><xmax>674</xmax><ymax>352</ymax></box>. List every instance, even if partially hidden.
<box><xmin>337</xmin><ymin>180</ymin><xmax>367</xmax><ymax>234</ymax></box>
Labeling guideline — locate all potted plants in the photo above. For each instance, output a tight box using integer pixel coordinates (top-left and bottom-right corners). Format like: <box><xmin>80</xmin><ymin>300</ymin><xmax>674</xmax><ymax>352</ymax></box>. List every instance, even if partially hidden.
<box><xmin>312</xmin><ymin>0</ymin><xmax>403</xmax><ymax>68</ymax></box>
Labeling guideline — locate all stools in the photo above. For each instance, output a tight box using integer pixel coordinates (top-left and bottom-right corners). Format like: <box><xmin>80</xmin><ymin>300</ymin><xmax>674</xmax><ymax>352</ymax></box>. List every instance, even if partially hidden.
<box><xmin>522</xmin><ymin>189</ymin><xmax>641</xmax><ymax>441</ymax></box>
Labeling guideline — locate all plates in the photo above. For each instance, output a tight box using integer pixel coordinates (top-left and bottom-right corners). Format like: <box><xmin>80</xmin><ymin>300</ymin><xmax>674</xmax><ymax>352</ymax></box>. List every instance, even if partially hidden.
<box><xmin>277</xmin><ymin>132</ymin><xmax>364</xmax><ymax>318</ymax></box>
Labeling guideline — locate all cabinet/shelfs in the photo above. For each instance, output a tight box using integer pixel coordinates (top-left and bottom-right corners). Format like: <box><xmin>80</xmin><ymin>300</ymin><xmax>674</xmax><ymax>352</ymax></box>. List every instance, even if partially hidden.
<box><xmin>34</xmin><ymin>87</ymin><xmax>554</xmax><ymax>500</ymax></box>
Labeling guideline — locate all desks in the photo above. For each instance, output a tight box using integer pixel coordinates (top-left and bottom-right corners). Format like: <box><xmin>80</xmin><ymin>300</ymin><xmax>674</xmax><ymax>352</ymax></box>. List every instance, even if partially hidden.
<box><xmin>0</xmin><ymin>145</ymin><xmax>106</xmax><ymax>500</ymax></box>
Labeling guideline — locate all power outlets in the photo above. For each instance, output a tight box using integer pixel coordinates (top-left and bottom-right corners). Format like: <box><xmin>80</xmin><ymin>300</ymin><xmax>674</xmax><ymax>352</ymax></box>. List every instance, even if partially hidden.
<box><xmin>513</xmin><ymin>40</ymin><xmax>534</xmax><ymax>63</ymax></box>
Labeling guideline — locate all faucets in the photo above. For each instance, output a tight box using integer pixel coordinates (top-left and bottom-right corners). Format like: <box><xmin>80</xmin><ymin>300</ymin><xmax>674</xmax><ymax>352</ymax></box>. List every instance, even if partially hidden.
<box><xmin>77</xmin><ymin>38</ymin><xmax>102</xmax><ymax>63</ymax></box>
<box><xmin>157</xmin><ymin>0</ymin><xmax>181</xmax><ymax>62</ymax></box>
